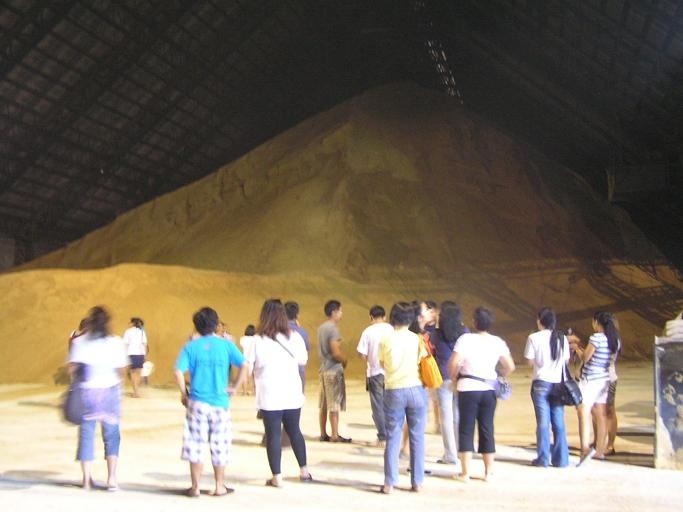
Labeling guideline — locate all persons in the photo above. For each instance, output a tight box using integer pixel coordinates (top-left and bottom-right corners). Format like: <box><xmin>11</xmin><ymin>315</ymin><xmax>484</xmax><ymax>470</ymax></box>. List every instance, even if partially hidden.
<box><xmin>240</xmin><ymin>298</ymin><xmax>313</xmax><ymax>487</ymax></box>
<box><xmin>67</xmin><ymin>306</ymin><xmax>148</xmax><ymax>490</ymax></box>
<box><xmin>590</xmin><ymin>313</ymin><xmax>622</xmax><ymax>456</ymax></box>
<box><xmin>356</xmin><ymin>298</ymin><xmax>470</xmax><ymax>492</ymax></box>
<box><xmin>565</xmin><ymin>311</ymin><xmax>618</xmax><ymax>467</ymax></box>
<box><xmin>174</xmin><ymin>306</ymin><xmax>248</xmax><ymax>497</ymax></box>
<box><xmin>447</xmin><ymin>306</ymin><xmax>515</xmax><ymax>481</ymax></box>
<box><xmin>316</xmin><ymin>299</ymin><xmax>353</xmax><ymax>442</ymax></box>
<box><xmin>524</xmin><ymin>306</ymin><xmax>571</xmax><ymax>468</ymax></box>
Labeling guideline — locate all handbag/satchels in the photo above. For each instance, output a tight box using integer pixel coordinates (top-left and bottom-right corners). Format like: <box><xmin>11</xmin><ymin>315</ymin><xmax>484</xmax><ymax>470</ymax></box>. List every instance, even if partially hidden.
<box><xmin>257</xmin><ymin>409</ymin><xmax>264</xmax><ymax>418</ymax></box>
<box><xmin>560</xmin><ymin>380</ymin><xmax>583</xmax><ymax>406</ymax></box>
<box><xmin>494</xmin><ymin>376</ymin><xmax>512</xmax><ymax>401</ymax></box>
<box><xmin>420</xmin><ymin>355</ymin><xmax>443</xmax><ymax>389</ymax></box>
<box><xmin>65</xmin><ymin>378</ymin><xmax>82</xmax><ymax>425</ymax></box>
